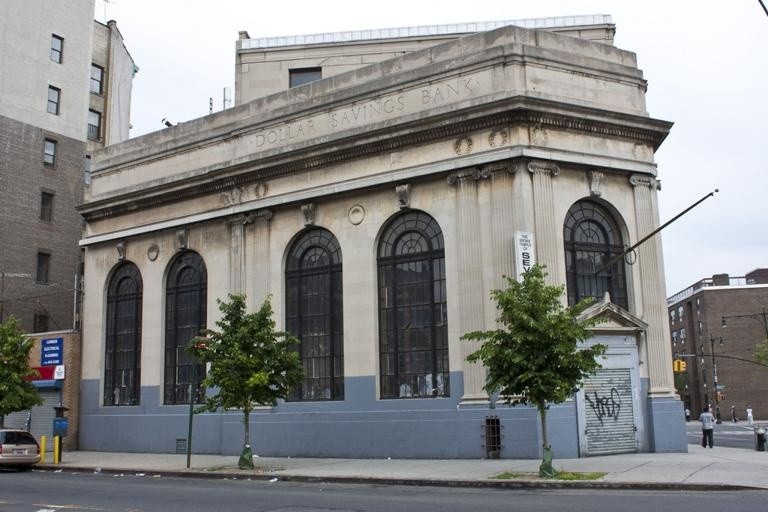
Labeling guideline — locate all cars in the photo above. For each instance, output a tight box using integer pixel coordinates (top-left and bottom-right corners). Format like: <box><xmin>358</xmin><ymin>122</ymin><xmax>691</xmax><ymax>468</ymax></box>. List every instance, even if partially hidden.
<box><xmin>0</xmin><ymin>428</ymin><xmax>41</xmax><ymax>472</ymax></box>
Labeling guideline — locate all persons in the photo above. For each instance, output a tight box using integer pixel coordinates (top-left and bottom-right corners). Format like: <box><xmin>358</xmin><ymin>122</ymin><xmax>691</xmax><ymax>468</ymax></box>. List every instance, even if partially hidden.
<box><xmin>746</xmin><ymin>404</ymin><xmax>754</xmax><ymax>425</ymax></box>
<box><xmin>685</xmin><ymin>406</ymin><xmax>691</xmax><ymax>422</ymax></box>
<box><xmin>698</xmin><ymin>407</ymin><xmax>715</xmax><ymax>448</ymax></box>
<box><xmin>731</xmin><ymin>406</ymin><xmax>738</xmax><ymax>423</ymax></box>
<box><xmin>707</xmin><ymin>403</ymin><xmax>713</xmax><ymax>414</ymax></box>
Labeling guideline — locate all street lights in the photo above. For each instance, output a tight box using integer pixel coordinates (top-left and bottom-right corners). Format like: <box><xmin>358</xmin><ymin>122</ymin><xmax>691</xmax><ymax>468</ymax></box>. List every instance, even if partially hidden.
<box><xmin>720</xmin><ymin>307</ymin><xmax>768</xmax><ymax>341</ymax></box>
<box><xmin>710</xmin><ymin>333</ymin><xmax>725</xmax><ymax>424</ymax></box>
